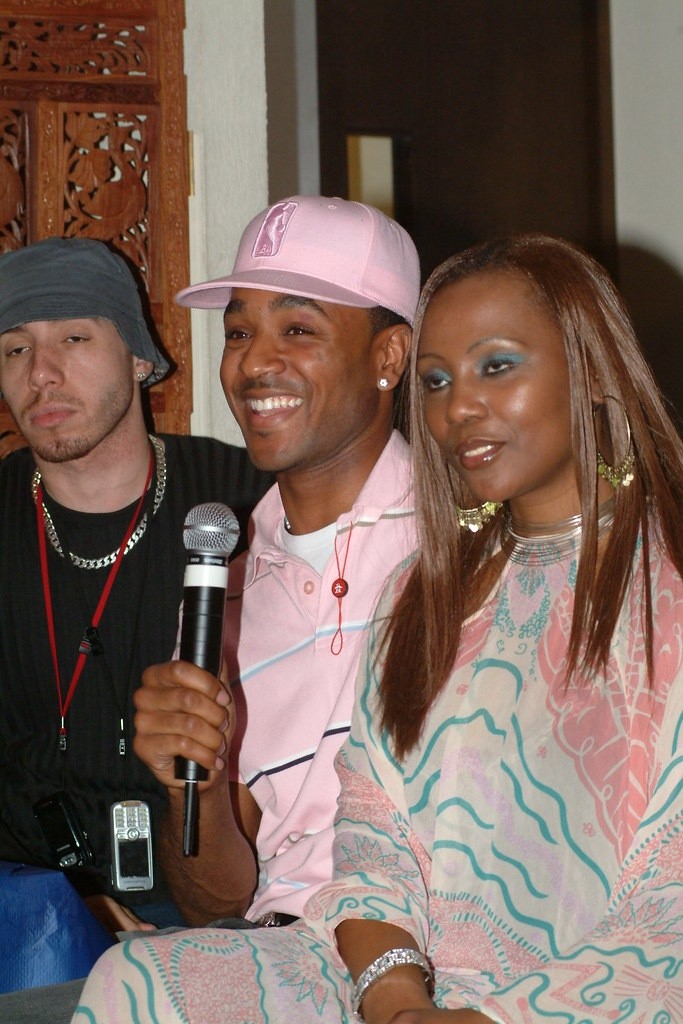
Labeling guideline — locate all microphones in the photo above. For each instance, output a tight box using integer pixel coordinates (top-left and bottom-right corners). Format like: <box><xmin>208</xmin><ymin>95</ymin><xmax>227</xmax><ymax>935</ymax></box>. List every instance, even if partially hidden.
<box><xmin>173</xmin><ymin>501</ymin><xmax>240</xmax><ymax>859</ymax></box>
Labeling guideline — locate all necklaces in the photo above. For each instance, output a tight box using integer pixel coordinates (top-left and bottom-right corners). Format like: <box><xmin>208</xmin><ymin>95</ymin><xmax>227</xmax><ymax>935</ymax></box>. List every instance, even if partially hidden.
<box><xmin>285</xmin><ymin>516</ymin><xmax>299</xmax><ymax>534</ymax></box>
<box><xmin>501</xmin><ymin>494</ymin><xmax>615</xmax><ymax>567</ymax></box>
<box><xmin>31</xmin><ymin>432</ymin><xmax>168</xmax><ymax>570</ymax></box>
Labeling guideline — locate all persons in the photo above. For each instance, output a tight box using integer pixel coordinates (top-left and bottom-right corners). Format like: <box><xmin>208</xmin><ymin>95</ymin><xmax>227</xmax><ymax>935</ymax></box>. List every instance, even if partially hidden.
<box><xmin>0</xmin><ymin>236</ymin><xmax>278</xmax><ymax>996</ymax></box>
<box><xmin>74</xmin><ymin>234</ymin><xmax>683</xmax><ymax>1024</ymax></box>
<box><xmin>0</xmin><ymin>194</ymin><xmax>425</xmax><ymax>1024</ymax></box>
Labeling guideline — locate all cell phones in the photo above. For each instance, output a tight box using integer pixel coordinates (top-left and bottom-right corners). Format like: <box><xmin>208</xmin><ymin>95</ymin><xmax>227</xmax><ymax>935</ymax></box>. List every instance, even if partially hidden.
<box><xmin>32</xmin><ymin>791</ymin><xmax>94</xmax><ymax>871</ymax></box>
<box><xmin>110</xmin><ymin>799</ymin><xmax>152</xmax><ymax>893</ymax></box>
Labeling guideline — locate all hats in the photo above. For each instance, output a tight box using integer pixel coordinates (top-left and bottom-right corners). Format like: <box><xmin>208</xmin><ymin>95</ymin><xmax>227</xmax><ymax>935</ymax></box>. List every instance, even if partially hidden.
<box><xmin>176</xmin><ymin>196</ymin><xmax>422</xmax><ymax>329</ymax></box>
<box><xmin>0</xmin><ymin>237</ymin><xmax>170</xmax><ymax>389</ymax></box>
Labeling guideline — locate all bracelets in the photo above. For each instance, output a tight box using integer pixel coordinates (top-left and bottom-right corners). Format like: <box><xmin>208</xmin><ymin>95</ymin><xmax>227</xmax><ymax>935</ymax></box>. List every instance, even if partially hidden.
<box><xmin>350</xmin><ymin>948</ymin><xmax>434</xmax><ymax>1015</ymax></box>
